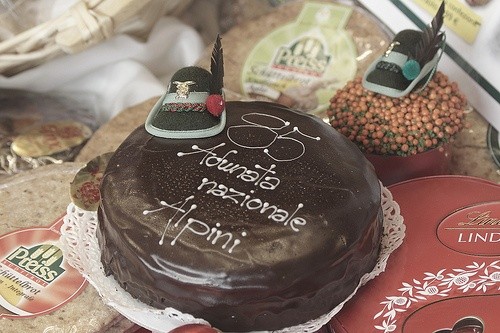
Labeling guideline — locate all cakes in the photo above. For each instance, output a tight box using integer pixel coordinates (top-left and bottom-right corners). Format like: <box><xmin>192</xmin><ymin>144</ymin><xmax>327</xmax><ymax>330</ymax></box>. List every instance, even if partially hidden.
<box><xmin>95</xmin><ymin>35</ymin><xmax>383</xmax><ymax>333</ymax></box>
<box><xmin>328</xmin><ymin>0</ymin><xmax>472</xmax><ymax>186</ymax></box>
